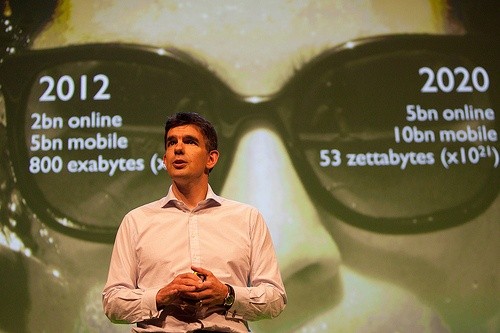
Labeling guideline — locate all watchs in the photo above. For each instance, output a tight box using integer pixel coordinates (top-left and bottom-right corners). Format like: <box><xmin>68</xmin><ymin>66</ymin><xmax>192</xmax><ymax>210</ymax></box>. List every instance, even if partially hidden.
<box><xmin>223</xmin><ymin>283</ymin><xmax>235</xmax><ymax>312</ymax></box>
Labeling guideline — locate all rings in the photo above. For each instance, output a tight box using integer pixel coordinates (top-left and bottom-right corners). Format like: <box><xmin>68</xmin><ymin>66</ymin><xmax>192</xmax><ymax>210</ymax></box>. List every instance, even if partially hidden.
<box><xmin>199</xmin><ymin>299</ymin><xmax>203</xmax><ymax>308</ymax></box>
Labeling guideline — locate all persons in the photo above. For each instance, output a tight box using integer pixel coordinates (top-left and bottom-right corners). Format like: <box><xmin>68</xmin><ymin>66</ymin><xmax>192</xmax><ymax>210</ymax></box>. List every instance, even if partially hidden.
<box><xmin>0</xmin><ymin>2</ymin><xmax>500</xmax><ymax>332</ymax></box>
<box><xmin>101</xmin><ymin>111</ymin><xmax>287</xmax><ymax>333</ymax></box>
